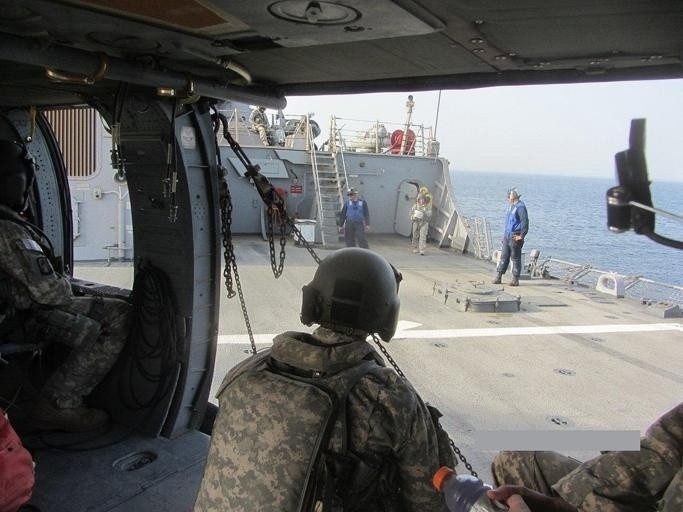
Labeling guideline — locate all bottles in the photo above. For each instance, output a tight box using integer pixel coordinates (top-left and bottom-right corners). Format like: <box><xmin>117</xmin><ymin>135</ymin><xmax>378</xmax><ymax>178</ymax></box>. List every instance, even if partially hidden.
<box><xmin>432</xmin><ymin>467</ymin><xmax>509</xmax><ymax>512</ymax></box>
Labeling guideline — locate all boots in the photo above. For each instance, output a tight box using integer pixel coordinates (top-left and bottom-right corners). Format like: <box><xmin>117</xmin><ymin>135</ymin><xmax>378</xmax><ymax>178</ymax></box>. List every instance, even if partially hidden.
<box><xmin>508</xmin><ymin>274</ymin><xmax>519</xmax><ymax>286</ymax></box>
<box><xmin>490</xmin><ymin>272</ymin><xmax>502</xmax><ymax>284</ymax></box>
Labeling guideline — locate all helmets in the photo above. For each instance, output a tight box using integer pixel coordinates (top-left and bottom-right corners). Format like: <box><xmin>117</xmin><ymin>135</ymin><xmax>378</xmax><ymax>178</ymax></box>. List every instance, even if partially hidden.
<box><xmin>301</xmin><ymin>247</ymin><xmax>403</xmax><ymax>343</ymax></box>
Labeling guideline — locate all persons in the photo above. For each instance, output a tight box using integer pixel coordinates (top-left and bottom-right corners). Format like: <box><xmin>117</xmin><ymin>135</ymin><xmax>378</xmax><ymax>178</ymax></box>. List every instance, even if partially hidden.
<box><xmin>409</xmin><ymin>193</ymin><xmax>432</xmax><ymax>255</ymax></box>
<box><xmin>1</xmin><ymin>138</ymin><xmax>132</xmax><ymax>425</ymax></box>
<box><xmin>249</xmin><ymin>106</ymin><xmax>281</xmax><ymax>146</ymax></box>
<box><xmin>490</xmin><ymin>402</ymin><xmax>683</xmax><ymax>512</ymax></box>
<box><xmin>190</xmin><ymin>246</ymin><xmax>454</xmax><ymax>510</ymax></box>
<box><xmin>338</xmin><ymin>187</ymin><xmax>370</xmax><ymax>248</ymax></box>
<box><xmin>491</xmin><ymin>187</ymin><xmax>529</xmax><ymax>286</ymax></box>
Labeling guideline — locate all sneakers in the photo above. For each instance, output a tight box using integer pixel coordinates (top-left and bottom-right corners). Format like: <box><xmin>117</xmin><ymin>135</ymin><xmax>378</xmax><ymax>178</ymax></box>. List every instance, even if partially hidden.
<box><xmin>411</xmin><ymin>247</ymin><xmax>426</xmax><ymax>255</ymax></box>
